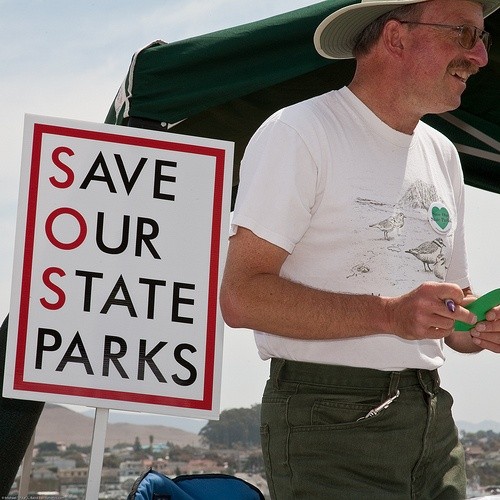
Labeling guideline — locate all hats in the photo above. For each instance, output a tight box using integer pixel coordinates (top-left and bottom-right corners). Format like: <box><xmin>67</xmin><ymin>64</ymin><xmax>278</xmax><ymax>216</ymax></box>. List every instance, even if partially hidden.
<box><xmin>313</xmin><ymin>0</ymin><xmax>500</xmax><ymax>60</ymax></box>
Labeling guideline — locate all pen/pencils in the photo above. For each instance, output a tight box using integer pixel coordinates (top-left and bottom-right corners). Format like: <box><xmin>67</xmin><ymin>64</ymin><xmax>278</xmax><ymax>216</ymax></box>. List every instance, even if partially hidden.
<box><xmin>444</xmin><ymin>298</ymin><xmax>456</xmax><ymax>313</ymax></box>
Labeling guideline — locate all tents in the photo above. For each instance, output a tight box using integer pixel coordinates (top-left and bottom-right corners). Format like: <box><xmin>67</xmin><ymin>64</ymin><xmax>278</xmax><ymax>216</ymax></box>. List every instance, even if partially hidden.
<box><xmin>0</xmin><ymin>0</ymin><xmax>500</xmax><ymax>500</ymax></box>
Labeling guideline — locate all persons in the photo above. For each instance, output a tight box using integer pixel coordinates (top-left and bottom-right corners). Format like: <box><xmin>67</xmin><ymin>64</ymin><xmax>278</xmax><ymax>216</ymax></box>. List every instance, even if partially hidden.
<box><xmin>219</xmin><ymin>0</ymin><xmax>500</xmax><ymax>500</ymax></box>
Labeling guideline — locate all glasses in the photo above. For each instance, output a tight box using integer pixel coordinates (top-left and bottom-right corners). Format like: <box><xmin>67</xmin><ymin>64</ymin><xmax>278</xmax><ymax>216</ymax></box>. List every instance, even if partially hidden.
<box><xmin>403</xmin><ymin>21</ymin><xmax>489</xmax><ymax>49</ymax></box>
